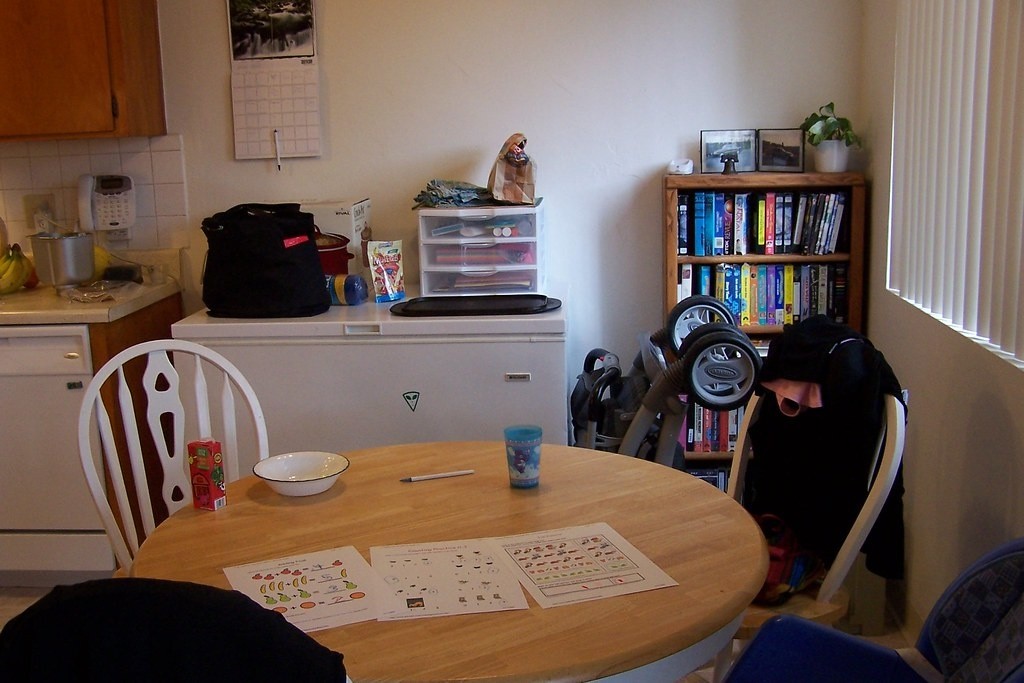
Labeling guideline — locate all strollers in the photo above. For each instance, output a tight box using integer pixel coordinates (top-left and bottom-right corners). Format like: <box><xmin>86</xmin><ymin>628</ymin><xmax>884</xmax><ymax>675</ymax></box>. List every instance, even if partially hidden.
<box><xmin>572</xmin><ymin>296</ymin><xmax>763</xmax><ymax>467</ymax></box>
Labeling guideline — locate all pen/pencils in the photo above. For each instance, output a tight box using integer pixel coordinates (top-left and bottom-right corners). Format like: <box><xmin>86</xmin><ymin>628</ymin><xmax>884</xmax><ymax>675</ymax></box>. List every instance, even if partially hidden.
<box><xmin>272</xmin><ymin>128</ymin><xmax>284</xmax><ymax>172</ymax></box>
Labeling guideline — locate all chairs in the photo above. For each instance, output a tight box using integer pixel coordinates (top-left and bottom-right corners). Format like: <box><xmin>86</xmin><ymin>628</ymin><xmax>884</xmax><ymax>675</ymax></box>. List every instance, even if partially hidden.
<box><xmin>78</xmin><ymin>339</ymin><xmax>271</xmax><ymax>564</ymax></box>
<box><xmin>723</xmin><ymin>539</ymin><xmax>1024</xmax><ymax>682</ymax></box>
<box><xmin>698</xmin><ymin>374</ymin><xmax>908</xmax><ymax>680</ymax></box>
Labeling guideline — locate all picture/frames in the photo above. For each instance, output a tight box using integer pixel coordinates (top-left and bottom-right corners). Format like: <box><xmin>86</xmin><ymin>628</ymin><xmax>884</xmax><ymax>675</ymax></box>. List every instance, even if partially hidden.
<box><xmin>755</xmin><ymin>128</ymin><xmax>807</xmax><ymax>173</ymax></box>
<box><xmin>700</xmin><ymin>129</ymin><xmax>757</xmax><ymax>174</ymax></box>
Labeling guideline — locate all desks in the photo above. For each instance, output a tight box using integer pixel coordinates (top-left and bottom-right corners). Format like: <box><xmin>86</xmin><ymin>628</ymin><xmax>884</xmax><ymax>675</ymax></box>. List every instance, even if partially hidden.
<box><xmin>128</xmin><ymin>440</ymin><xmax>767</xmax><ymax>680</ymax></box>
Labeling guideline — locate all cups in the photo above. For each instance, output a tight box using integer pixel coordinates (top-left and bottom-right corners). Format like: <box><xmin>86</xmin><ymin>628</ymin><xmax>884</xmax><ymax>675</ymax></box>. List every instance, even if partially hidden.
<box><xmin>503</xmin><ymin>425</ymin><xmax>543</xmax><ymax>489</ymax></box>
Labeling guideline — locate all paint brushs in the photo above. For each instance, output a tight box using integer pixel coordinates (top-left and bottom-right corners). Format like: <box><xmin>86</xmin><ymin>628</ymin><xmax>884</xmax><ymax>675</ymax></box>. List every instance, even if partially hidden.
<box><xmin>399</xmin><ymin>470</ymin><xmax>476</xmax><ymax>482</ymax></box>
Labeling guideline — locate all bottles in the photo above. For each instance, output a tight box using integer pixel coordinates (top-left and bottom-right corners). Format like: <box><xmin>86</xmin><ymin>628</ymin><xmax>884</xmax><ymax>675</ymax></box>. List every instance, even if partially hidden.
<box><xmin>322</xmin><ymin>272</ymin><xmax>369</xmax><ymax>305</ymax></box>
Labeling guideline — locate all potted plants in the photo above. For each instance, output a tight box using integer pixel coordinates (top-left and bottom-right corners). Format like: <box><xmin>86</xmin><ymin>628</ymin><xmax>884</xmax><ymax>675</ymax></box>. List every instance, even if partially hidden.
<box><xmin>797</xmin><ymin>103</ymin><xmax>863</xmax><ymax>173</ymax></box>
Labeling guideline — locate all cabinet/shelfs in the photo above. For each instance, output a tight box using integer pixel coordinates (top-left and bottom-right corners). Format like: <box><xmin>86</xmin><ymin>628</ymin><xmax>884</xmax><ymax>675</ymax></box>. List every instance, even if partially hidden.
<box><xmin>419</xmin><ymin>195</ymin><xmax>548</xmax><ymax>297</ymax></box>
<box><xmin>0</xmin><ymin>2</ymin><xmax>164</xmax><ymax>145</ymax></box>
<box><xmin>664</xmin><ymin>170</ymin><xmax>868</xmax><ymax>464</ymax></box>
<box><xmin>2</xmin><ymin>286</ymin><xmax>187</xmax><ymax>594</ymax></box>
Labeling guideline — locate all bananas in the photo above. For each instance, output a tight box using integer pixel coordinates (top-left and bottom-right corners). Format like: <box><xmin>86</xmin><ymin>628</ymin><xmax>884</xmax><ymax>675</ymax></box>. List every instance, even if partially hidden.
<box><xmin>0</xmin><ymin>243</ymin><xmax>33</xmax><ymax>295</ymax></box>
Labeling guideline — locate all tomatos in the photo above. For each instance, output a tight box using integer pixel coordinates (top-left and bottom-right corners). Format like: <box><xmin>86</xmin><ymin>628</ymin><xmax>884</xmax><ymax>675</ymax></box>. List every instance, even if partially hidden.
<box><xmin>22</xmin><ymin>267</ymin><xmax>39</xmax><ymax>289</ymax></box>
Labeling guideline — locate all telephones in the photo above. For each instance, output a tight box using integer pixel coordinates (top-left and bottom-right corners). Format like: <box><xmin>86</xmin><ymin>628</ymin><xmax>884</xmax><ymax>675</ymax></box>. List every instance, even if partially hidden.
<box><xmin>76</xmin><ymin>170</ymin><xmax>138</xmax><ymax>234</ymax></box>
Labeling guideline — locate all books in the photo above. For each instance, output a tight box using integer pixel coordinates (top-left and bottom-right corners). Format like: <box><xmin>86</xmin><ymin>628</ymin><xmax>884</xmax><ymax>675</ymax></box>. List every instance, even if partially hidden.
<box><xmin>673</xmin><ymin>182</ymin><xmax>851</xmax><ymax>495</ymax></box>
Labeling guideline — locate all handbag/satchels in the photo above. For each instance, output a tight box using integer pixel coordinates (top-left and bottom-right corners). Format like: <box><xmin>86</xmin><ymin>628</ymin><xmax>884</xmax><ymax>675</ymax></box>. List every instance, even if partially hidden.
<box><xmin>201</xmin><ymin>201</ymin><xmax>334</xmax><ymax>318</ymax></box>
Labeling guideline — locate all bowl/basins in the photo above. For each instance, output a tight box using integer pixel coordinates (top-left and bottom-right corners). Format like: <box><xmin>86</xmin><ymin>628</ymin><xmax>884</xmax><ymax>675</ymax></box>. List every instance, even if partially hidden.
<box><xmin>28</xmin><ymin>231</ymin><xmax>95</xmax><ymax>286</ymax></box>
<box><xmin>315</xmin><ymin>229</ymin><xmax>356</xmax><ymax>277</ymax></box>
<box><xmin>253</xmin><ymin>450</ymin><xmax>350</xmax><ymax>497</ymax></box>
